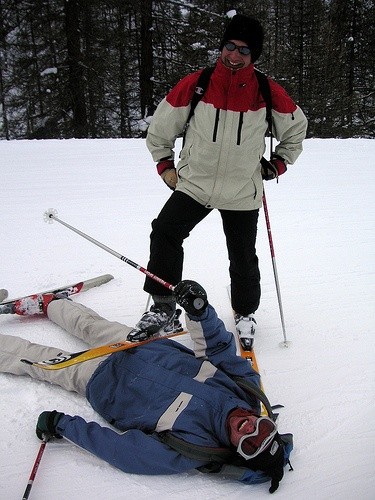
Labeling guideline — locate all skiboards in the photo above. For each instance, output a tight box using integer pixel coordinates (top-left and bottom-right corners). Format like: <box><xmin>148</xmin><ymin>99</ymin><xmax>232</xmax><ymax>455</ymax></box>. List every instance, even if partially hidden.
<box><xmin>19</xmin><ymin>282</ymin><xmax>271</xmax><ymax>419</ymax></box>
<box><xmin>0</xmin><ymin>272</ymin><xmax>116</xmax><ymax>317</ymax></box>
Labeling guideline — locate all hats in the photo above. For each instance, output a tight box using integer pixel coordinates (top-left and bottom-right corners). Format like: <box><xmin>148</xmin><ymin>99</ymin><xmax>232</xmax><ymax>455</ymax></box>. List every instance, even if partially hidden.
<box><xmin>220</xmin><ymin>15</ymin><xmax>263</xmax><ymax>63</ymax></box>
<box><xmin>249</xmin><ymin>432</ymin><xmax>285</xmax><ymax>472</ymax></box>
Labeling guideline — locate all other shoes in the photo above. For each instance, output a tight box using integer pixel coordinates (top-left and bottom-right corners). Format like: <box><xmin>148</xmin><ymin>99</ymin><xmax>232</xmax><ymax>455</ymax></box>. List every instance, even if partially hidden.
<box><xmin>14</xmin><ymin>293</ymin><xmax>55</xmax><ymax>314</ymax></box>
<box><xmin>234</xmin><ymin>308</ymin><xmax>255</xmax><ymax>348</ymax></box>
<box><xmin>142</xmin><ymin>301</ymin><xmax>175</xmax><ymax>334</ymax></box>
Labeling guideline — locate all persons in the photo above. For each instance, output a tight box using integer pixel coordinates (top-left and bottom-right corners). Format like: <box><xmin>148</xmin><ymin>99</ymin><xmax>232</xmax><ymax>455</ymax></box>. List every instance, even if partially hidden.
<box><xmin>126</xmin><ymin>14</ymin><xmax>310</xmax><ymax>350</ymax></box>
<box><xmin>0</xmin><ymin>280</ymin><xmax>294</xmax><ymax>494</ymax></box>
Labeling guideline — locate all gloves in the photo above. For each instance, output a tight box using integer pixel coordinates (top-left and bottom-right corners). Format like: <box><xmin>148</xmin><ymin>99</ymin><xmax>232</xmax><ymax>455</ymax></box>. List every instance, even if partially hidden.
<box><xmin>261</xmin><ymin>158</ymin><xmax>287</xmax><ymax>181</ymax></box>
<box><xmin>157</xmin><ymin>160</ymin><xmax>176</xmax><ymax>190</ymax></box>
<box><xmin>36</xmin><ymin>410</ymin><xmax>65</xmax><ymax>439</ymax></box>
<box><xmin>174</xmin><ymin>281</ymin><xmax>208</xmax><ymax>315</ymax></box>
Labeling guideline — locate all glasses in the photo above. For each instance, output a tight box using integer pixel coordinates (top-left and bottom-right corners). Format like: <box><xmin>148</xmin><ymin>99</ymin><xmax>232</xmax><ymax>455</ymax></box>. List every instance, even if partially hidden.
<box><xmin>224</xmin><ymin>41</ymin><xmax>251</xmax><ymax>56</ymax></box>
<box><xmin>236</xmin><ymin>418</ymin><xmax>276</xmax><ymax>461</ymax></box>
